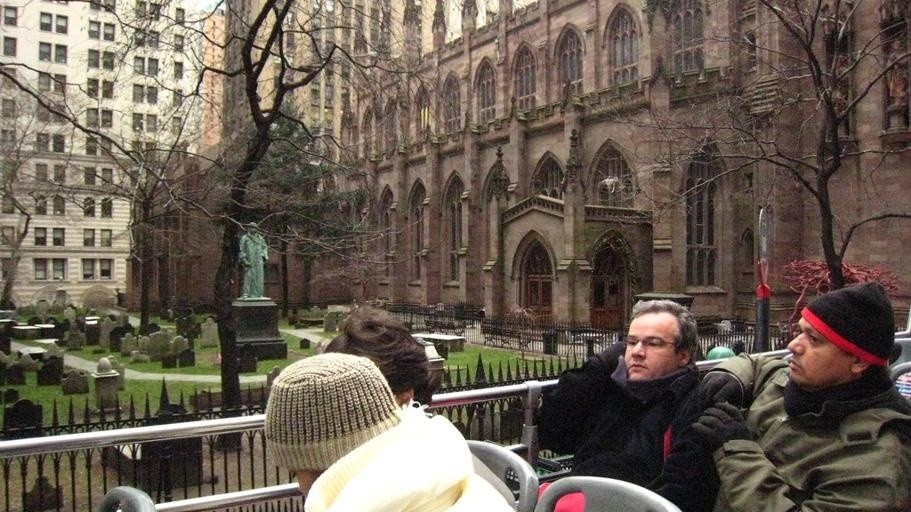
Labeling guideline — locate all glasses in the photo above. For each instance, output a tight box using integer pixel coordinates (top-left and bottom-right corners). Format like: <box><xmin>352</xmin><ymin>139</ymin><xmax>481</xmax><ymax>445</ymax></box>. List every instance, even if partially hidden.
<box><xmin>623</xmin><ymin>335</ymin><xmax>677</xmax><ymax>349</ymax></box>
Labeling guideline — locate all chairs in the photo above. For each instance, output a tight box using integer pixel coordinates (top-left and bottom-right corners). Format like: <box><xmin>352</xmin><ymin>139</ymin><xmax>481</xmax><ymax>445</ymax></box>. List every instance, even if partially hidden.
<box><xmin>466</xmin><ymin>437</ymin><xmax>685</xmax><ymax>512</ymax></box>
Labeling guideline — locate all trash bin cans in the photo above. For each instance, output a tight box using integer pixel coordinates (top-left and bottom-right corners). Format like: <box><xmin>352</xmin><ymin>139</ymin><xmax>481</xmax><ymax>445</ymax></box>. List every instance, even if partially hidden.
<box><xmin>542</xmin><ymin>333</ymin><xmax>557</xmax><ymax>354</ymax></box>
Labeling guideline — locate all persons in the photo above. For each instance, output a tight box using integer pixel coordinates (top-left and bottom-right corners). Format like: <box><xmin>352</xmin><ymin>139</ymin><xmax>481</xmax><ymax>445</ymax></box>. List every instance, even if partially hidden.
<box><xmin>238</xmin><ymin>221</ymin><xmax>269</xmax><ymax>298</ymax></box>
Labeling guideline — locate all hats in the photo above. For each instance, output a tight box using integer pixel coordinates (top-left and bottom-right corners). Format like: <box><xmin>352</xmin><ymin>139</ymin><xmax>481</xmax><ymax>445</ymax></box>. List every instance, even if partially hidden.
<box><xmin>801</xmin><ymin>282</ymin><xmax>895</xmax><ymax>366</ymax></box>
<box><xmin>264</xmin><ymin>352</ymin><xmax>401</xmax><ymax>470</ymax></box>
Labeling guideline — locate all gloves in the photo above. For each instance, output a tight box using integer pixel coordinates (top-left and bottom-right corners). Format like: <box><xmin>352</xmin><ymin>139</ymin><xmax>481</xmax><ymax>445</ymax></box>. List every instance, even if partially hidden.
<box><xmin>589</xmin><ymin>342</ymin><xmax>627</xmax><ymax>369</ymax></box>
<box><xmin>691</xmin><ymin>402</ymin><xmax>751</xmax><ymax>448</ymax></box>
<box><xmin>697</xmin><ymin>372</ymin><xmax>741</xmax><ymax>404</ymax></box>
